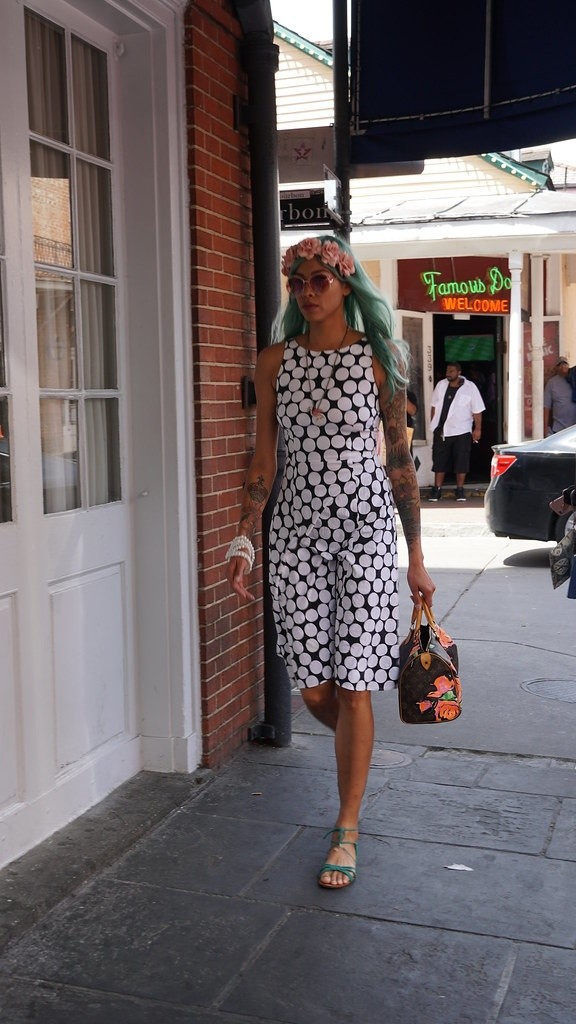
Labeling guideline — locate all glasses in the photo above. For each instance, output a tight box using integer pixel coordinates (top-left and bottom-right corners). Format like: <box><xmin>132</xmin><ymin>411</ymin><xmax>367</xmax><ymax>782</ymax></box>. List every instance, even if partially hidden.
<box><xmin>286</xmin><ymin>273</ymin><xmax>336</xmax><ymax>296</ymax></box>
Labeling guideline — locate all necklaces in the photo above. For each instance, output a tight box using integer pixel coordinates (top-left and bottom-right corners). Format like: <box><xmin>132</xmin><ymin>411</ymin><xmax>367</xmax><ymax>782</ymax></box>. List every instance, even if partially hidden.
<box><xmin>306</xmin><ymin>327</ymin><xmax>348</xmax><ymax>415</ymax></box>
<box><xmin>450</xmin><ymin>380</ymin><xmax>460</xmax><ymax>391</ymax></box>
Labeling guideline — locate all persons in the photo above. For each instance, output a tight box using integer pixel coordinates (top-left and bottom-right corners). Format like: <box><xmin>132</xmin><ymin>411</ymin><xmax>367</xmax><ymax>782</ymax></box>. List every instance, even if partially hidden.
<box><xmin>405</xmin><ymin>388</ymin><xmax>419</xmax><ymax>458</ymax></box>
<box><xmin>544</xmin><ymin>358</ymin><xmax>576</xmax><ymax>439</ymax></box>
<box><xmin>428</xmin><ymin>362</ymin><xmax>487</xmax><ymax>502</ymax></box>
<box><xmin>225</xmin><ymin>236</ymin><xmax>436</xmax><ymax>892</ymax></box>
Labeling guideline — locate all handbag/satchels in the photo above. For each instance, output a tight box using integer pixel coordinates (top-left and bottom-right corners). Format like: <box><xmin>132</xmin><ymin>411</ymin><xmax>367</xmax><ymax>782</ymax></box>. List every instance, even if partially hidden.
<box><xmin>397</xmin><ymin>594</ymin><xmax>463</xmax><ymax>725</ymax></box>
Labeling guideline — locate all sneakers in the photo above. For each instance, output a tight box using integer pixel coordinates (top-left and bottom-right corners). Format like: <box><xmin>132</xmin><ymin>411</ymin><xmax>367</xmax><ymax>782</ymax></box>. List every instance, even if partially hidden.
<box><xmin>454</xmin><ymin>487</ymin><xmax>467</xmax><ymax>502</ymax></box>
<box><xmin>428</xmin><ymin>485</ymin><xmax>441</xmax><ymax>502</ymax></box>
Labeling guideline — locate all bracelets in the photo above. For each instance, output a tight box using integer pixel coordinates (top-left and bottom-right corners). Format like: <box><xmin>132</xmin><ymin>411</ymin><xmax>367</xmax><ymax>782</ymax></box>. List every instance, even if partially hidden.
<box><xmin>225</xmin><ymin>534</ymin><xmax>256</xmax><ymax>574</ymax></box>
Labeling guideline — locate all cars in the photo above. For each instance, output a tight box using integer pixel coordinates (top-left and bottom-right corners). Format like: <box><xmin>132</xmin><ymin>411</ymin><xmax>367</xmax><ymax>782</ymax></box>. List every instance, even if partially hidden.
<box><xmin>484</xmin><ymin>423</ymin><xmax>576</xmax><ymax>542</ymax></box>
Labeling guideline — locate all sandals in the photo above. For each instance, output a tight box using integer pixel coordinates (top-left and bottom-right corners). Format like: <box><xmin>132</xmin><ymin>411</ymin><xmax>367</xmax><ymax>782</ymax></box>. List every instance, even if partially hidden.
<box><xmin>317</xmin><ymin>826</ymin><xmax>359</xmax><ymax>888</ymax></box>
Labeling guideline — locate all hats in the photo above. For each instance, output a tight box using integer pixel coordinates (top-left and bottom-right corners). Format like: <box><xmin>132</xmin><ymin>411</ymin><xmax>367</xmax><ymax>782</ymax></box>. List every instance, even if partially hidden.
<box><xmin>556</xmin><ymin>356</ymin><xmax>570</xmax><ymax>366</ymax></box>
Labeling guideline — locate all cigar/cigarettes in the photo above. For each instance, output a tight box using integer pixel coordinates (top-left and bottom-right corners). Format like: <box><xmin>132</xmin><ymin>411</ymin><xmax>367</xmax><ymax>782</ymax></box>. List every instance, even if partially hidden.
<box><xmin>475</xmin><ymin>440</ymin><xmax>478</xmax><ymax>443</ymax></box>
